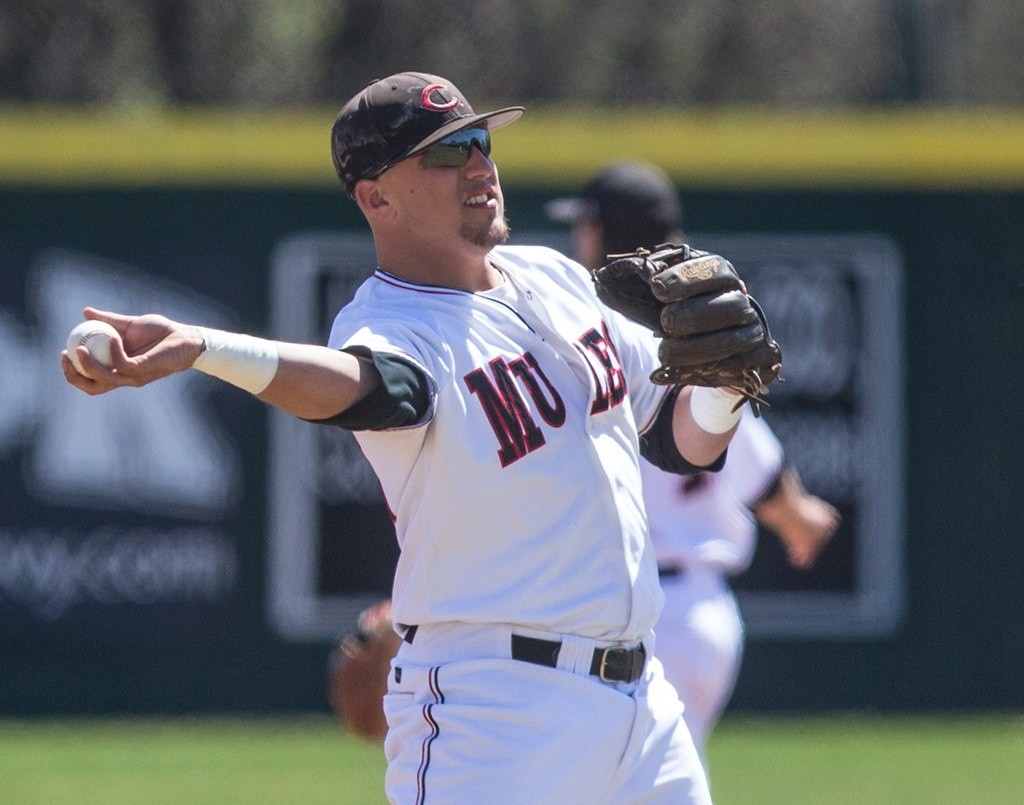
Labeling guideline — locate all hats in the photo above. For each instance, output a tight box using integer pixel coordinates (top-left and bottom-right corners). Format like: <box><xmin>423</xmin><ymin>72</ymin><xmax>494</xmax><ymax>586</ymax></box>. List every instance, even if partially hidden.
<box><xmin>546</xmin><ymin>161</ymin><xmax>682</xmax><ymax>236</ymax></box>
<box><xmin>331</xmin><ymin>72</ymin><xmax>526</xmax><ymax>202</ymax></box>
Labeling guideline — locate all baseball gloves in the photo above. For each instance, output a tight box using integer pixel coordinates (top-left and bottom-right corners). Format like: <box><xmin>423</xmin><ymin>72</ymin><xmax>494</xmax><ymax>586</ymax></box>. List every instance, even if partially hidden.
<box><xmin>331</xmin><ymin>600</ymin><xmax>405</xmax><ymax>740</ymax></box>
<box><xmin>590</xmin><ymin>240</ymin><xmax>787</xmax><ymax>418</ymax></box>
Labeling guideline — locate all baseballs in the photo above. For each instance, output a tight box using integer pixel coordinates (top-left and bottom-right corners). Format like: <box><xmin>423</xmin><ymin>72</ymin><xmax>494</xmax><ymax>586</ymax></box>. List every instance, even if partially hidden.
<box><xmin>65</xmin><ymin>319</ymin><xmax>124</xmax><ymax>383</ymax></box>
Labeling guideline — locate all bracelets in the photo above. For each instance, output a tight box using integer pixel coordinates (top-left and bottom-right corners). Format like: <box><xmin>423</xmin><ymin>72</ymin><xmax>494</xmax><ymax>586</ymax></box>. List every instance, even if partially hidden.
<box><xmin>194</xmin><ymin>317</ymin><xmax>279</xmax><ymax>398</ymax></box>
<box><xmin>690</xmin><ymin>379</ymin><xmax>747</xmax><ymax>435</ymax></box>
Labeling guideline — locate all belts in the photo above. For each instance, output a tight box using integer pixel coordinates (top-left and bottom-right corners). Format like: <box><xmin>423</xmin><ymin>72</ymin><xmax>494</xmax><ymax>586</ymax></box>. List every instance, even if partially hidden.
<box><xmin>405</xmin><ymin>626</ymin><xmax>644</xmax><ymax>684</ymax></box>
<box><xmin>660</xmin><ymin>569</ymin><xmax>732</xmax><ymax>590</ymax></box>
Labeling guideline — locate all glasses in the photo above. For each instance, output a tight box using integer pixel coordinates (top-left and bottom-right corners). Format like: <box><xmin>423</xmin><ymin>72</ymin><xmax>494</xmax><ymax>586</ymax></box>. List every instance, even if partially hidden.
<box><xmin>348</xmin><ymin>124</ymin><xmax>491</xmax><ymax>196</ymax></box>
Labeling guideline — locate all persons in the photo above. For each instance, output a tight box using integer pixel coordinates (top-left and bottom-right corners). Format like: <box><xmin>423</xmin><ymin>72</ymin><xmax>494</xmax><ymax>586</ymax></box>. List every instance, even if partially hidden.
<box><xmin>61</xmin><ymin>73</ymin><xmax>781</xmax><ymax>805</ymax></box>
<box><xmin>329</xmin><ymin>159</ymin><xmax>839</xmax><ymax>765</ymax></box>
